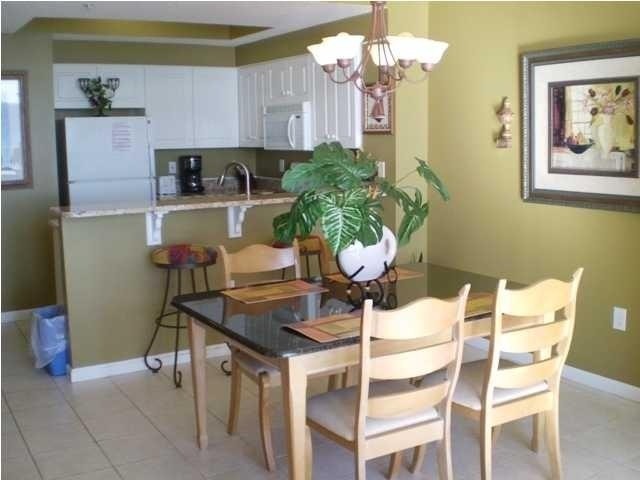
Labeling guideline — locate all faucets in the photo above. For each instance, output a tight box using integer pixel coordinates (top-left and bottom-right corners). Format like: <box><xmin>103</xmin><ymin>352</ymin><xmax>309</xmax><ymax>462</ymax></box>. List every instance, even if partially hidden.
<box><xmin>218</xmin><ymin>161</ymin><xmax>252</xmax><ymax>199</ymax></box>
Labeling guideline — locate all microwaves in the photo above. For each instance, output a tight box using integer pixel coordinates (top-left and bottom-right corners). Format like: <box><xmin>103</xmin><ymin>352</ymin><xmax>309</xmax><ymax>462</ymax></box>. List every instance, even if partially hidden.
<box><xmin>263</xmin><ymin>104</ymin><xmax>311</xmax><ymax>151</ymax></box>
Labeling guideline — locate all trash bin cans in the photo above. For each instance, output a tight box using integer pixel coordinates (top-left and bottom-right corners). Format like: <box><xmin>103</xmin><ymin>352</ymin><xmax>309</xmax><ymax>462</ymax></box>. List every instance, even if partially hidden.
<box><xmin>30</xmin><ymin>303</ymin><xmax>70</xmax><ymax>376</ymax></box>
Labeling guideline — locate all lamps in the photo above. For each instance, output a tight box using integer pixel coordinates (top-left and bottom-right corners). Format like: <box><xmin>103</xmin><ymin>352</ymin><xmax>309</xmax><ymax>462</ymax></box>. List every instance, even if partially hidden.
<box><xmin>306</xmin><ymin>1</ymin><xmax>450</xmax><ymax>124</ymax></box>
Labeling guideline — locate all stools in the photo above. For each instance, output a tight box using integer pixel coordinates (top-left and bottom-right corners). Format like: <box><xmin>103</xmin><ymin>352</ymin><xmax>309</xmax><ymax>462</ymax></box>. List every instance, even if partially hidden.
<box><xmin>143</xmin><ymin>241</ymin><xmax>234</xmax><ymax>387</ymax></box>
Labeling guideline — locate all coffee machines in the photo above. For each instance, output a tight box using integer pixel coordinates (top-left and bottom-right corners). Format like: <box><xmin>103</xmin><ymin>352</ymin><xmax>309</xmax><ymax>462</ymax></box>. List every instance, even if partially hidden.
<box><xmin>179</xmin><ymin>155</ymin><xmax>204</xmax><ymax>194</ymax></box>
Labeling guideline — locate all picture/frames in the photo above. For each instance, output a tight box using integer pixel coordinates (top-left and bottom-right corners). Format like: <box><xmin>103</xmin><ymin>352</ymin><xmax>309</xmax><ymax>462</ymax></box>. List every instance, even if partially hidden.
<box><xmin>1</xmin><ymin>69</ymin><xmax>36</xmax><ymax>189</ymax></box>
<box><xmin>360</xmin><ymin>81</ymin><xmax>395</xmax><ymax>134</ymax></box>
<box><xmin>517</xmin><ymin>37</ymin><xmax>640</xmax><ymax>214</ymax></box>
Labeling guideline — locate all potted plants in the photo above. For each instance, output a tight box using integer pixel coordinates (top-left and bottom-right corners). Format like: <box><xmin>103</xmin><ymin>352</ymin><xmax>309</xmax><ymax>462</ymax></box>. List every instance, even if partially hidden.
<box><xmin>271</xmin><ymin>136</ymin><xmax>449</xmax><ymax>282</ymax></box>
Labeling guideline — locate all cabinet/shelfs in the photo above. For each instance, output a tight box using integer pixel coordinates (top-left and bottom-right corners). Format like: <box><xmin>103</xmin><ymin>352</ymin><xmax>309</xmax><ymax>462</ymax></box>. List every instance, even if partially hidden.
<box><xmin>307</xmin><ymin>52</ymin><xmax>363</xmax><ymax>150</ymax></box>
<box><xmin>265</xmin><ymin>55</ymin><xmax>309</xmax><ymax>107</ymax></box>
<box><xmin>237</xmin><ymin>61</ymin><xmax>266</xmax><ymax>148</ymax></box>
<box><xmin>54</xmin><ymin>65</ymin><xmax>239</xmax><ymax>149</ymax></box>
